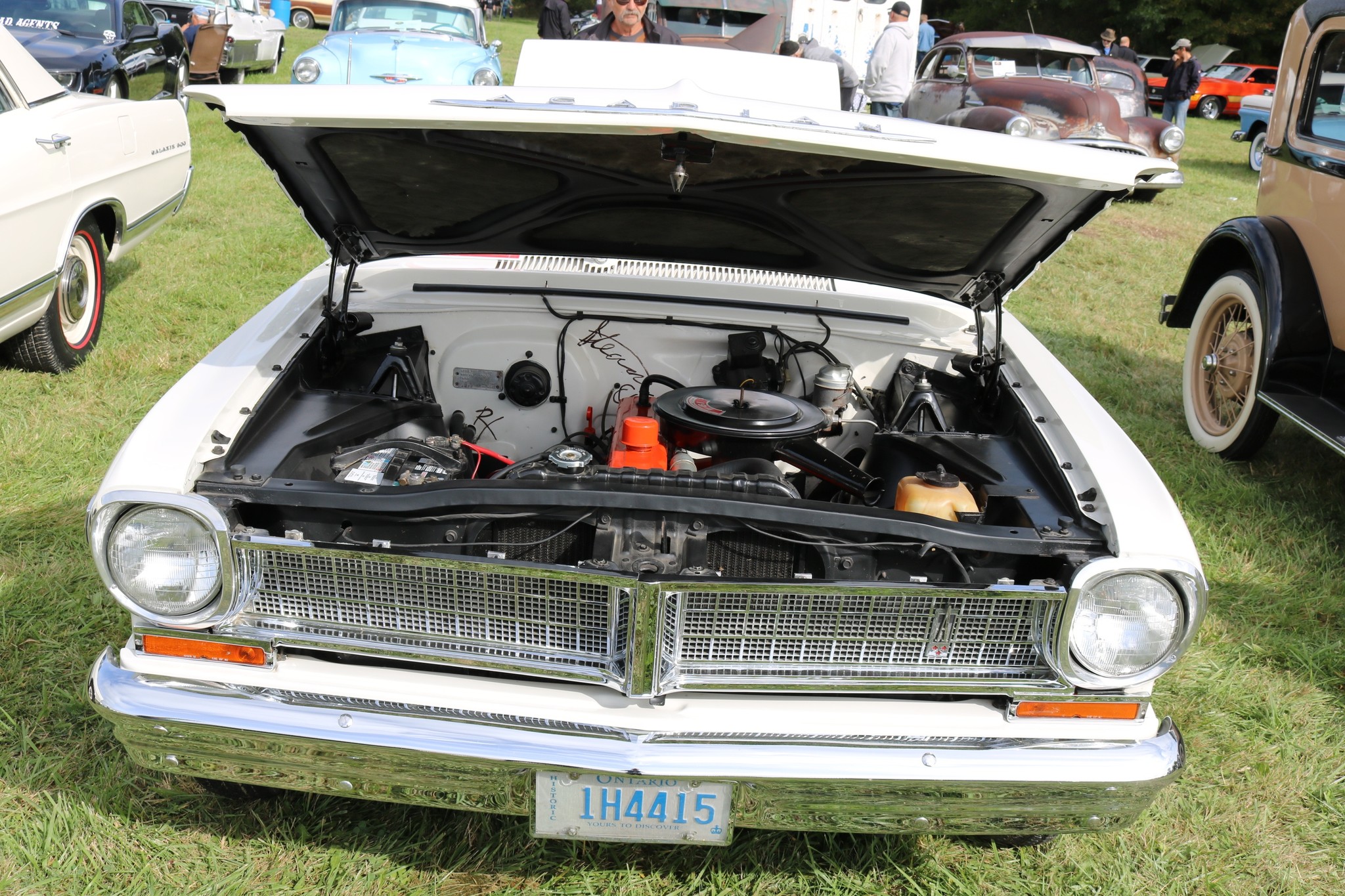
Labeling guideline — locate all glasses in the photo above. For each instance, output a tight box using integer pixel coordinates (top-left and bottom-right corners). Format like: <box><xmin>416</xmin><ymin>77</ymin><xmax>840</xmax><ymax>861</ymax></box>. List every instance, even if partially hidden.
<box><xmin>616</xmin><ymin>0</ymin><xmax>647</xmax><ymax>5</ymax></box>
<box><xmin>1102</xmin><ymin>39</ymin><xmax>1110</xmax><ymax>42</ymax></box>
<box><xmin>888</xmin><ymin>12</ymin><xmax>891</xmax><ymax>15</ymax></box>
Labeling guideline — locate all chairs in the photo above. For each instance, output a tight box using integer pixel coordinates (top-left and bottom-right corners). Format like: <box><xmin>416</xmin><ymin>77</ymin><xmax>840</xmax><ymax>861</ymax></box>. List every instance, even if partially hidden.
<box><xmin>190</xmin><ymin>24</ymin><xmax>233</xmax><ymax>84</ymax></box>
<box><xmin>93</xmin><ymin>9</ymin><xmax>109</xmax><ymax>29</ymax></box>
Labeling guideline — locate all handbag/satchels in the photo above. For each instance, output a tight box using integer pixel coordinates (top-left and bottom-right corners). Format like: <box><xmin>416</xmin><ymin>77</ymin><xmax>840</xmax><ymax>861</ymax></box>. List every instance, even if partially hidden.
<box><xmin>509</xmin><ymin>8</ymin><xmax>513</xmax><ymax>17</ymax></box>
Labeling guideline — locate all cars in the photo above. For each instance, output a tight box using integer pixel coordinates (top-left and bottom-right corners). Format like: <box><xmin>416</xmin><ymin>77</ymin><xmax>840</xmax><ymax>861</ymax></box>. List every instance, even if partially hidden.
<box><xmin>1155</xmin><ymin>0</ymin><xmax>1344</xmax><ymax>462</ymax></box>
<box><xmin>0</xmin><ymin>0</ymin><xmax>360</xmax><ymax>375</ymax></box>
<box><xmin>898</xmin><ymin>18</ymin><xmax>1345</xmax><ymax>203</ymax></box>
<box><xmin>88</xmin><ymin>39</ymin><xmax>1203</xmax><ymax>848</ymax></box>
<box><xmin>290</xmin><ymin>0</ymin><xmax>502</xmax><ymax>86</ymax></box>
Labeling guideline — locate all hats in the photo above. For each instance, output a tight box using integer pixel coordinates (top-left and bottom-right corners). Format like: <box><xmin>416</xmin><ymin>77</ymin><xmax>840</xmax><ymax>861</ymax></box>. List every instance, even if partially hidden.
<box><xmin>188</xmin><ymin>5</ymin><xmax>210</xmax><ymax>17</ymax></box>
<box><xmin>887</xmin><ymin>1</ymin><xmax>910</xmax><ymax>17</ymax></box>
<box><xmin>1171</xmin><ymin>38</ymin><xmax>1192</xmax><ymax>51</ymax></box>
<box><xmin>780</xmin><ymin>41</ymin><xmax>799</xmax><ymax>56</ymax></box>
<box><xmin>1100</xmin><ymin>28</ymin><xmax>1117</xmax><ymax>41</ymax></box>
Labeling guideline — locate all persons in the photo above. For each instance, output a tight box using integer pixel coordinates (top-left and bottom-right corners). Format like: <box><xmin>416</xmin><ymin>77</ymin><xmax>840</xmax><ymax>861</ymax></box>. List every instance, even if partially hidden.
<box><xmin>916</xmin><ymin>14</ymin><xmax>935</xmax><ymax>70</ymax></box>
<box><xmin>1162</xmin><ymin>38</ymin><xmax>1201</xmax><ymax>131</ymax></box>
<box><xmin>863</xmin><ymin>1</ymin><xmax>916</xmax><ymax>119</ymax></box>
<box><xmin>572</xmin><ymin>0</ymin><xmax>683</xmax><ymax>45</ymax></box>
<box><xmin>1090</xmin><ymin>27</ymin><xmax>1120</xmax><ymax>58</ymax></box>
<box><xmin>778</xmin><ymin>37</ymin><xmax>859</xmax><ymax>111</ymax></box>
<box><xmin>1120</xmin><ymin>36</ymin><xmax>1140</xmax><ymax>67</ymax></box>
<box><xmin>949</xmin><ymin>21</ymin><xmax>964</xmax><ymax>35</ymax></box>
<box><xmin>479</xmin><ymin>0</ymin><xmax>514</xmax><ymax>22</ymax></box>
<box><xmin>537</xmin><ymin>0</ymin><xmax>574</xmax><ymax>39</ymax></box>
<box><xmin>180</xmin><ymin>5</ymin><xmax>210</xmax><ymax>80</ymax></box>
